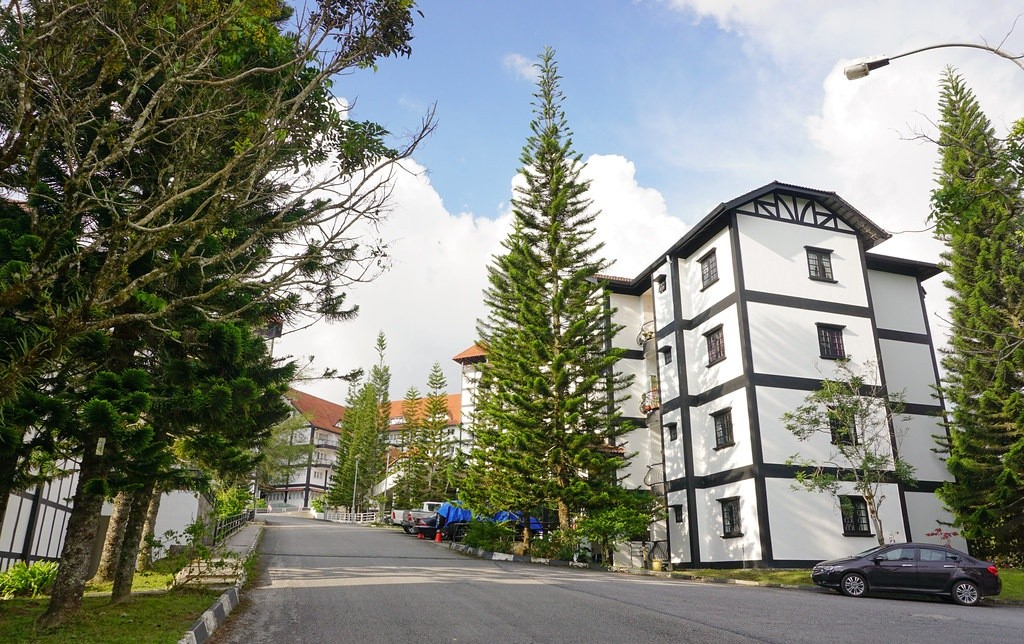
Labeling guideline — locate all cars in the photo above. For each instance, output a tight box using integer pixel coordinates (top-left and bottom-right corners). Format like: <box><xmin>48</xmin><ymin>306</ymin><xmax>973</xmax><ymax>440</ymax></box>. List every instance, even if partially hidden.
<box><xmin>808</xmin><ymin>541</ymin><xmax>1002</xmax><ymax>606</ymax></box>
<box><xmin>412</xmin><ymin>512</ymin><xmax>438</xmax><ymax>540</ymax></box>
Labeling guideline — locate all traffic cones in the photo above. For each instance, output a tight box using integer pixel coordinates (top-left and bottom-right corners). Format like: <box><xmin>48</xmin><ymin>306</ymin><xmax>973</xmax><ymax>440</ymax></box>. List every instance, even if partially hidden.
<box><xmin>435</xmin><ymin>531</ymin><xmax>443</xmax><ymax>544</ymax></box>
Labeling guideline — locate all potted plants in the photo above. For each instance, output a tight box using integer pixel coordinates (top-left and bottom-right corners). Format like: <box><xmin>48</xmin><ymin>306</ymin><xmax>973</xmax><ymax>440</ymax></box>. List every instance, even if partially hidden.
<box><xmin>643</xmin><ymin>330</ymin><xmax>653</xmax><ymax>341</ymax></box>
<box><xmin>642</xmin><ymin>399</ymin><xmax>660</xmax><ymax>411</ymax></box>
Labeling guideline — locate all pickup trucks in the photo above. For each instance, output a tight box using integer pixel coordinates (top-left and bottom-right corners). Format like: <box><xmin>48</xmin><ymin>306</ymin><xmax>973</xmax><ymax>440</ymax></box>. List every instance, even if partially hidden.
<box><xmin>390</xmin><ymin>501</ymin><xmax>445</xmax><ymax>535</ymax></box>
